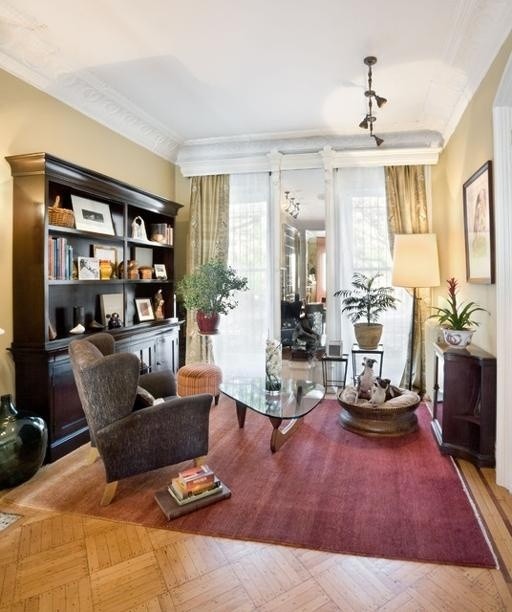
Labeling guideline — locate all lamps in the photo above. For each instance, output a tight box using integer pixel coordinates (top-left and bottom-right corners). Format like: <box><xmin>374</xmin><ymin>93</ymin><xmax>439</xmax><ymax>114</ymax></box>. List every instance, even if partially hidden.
<box><xmin>359</xmin><ymin>56</ymin><xmax>387</xmax><ymax>146</ymax></box>
<box><xmin>284</xmin><ymin>192</ymin><xmax>300</xmax><ymax>220</ymax></box>
<box><xmin>390</xmin><ymin>235</ymin><xmax>443</xmax><ymax>395</ymax></box>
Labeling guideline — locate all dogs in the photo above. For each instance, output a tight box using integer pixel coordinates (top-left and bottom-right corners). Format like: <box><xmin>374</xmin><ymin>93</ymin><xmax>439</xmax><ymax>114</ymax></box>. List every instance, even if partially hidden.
<box><xmin>368</xmin><ymin>377</ymin><xmax>402</xmax><ymax>405</ymax></box>
<box><xmin>354</xmin><ymin>356</ymin><xmax>377</xmax><ymax>404</ymax></box>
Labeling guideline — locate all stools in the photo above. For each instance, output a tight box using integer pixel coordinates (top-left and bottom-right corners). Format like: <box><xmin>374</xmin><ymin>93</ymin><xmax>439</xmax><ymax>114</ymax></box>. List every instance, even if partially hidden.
<box><xmin>176</xmin><ymin>363</ymin><xmax>221</xmax><ymax>408</ymax></box>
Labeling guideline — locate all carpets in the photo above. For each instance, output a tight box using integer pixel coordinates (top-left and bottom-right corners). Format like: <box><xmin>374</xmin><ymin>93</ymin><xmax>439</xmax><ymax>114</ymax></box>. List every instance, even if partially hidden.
<box><xmin>3</xmin><ymin>376</ymin><xmax>497</xmax><ymax>571</ymax></box>
<box><xmin>282</xmin><ymin>346</ymin><xmax>326</xmax><ymax>361</ymax></box>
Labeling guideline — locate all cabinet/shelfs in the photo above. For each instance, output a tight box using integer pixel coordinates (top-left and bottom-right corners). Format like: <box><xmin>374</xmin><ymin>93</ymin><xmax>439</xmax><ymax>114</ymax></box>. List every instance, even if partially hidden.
<box><xmin>195</xmin><ymin>331</ymin><xmax>219</xmax><ymax>364</ymax></box>
<box><xmin>282</xmin><ymin>223</ymin><xmax>299</xmax><ymax>302</ymax></box>
<box><xmin>428</xmin><ymin>334</ymin><xmax>498</xmax><ymax>468</ymax></box>
<box><xmin>350</xmin><ymin>345</ymin><xmax>386</xmax><ymax>389</ymax></box>
<box><xmin>321</xmin><ymin>354</ymin><xmax>350</xmax><ymax>395</ymax></box>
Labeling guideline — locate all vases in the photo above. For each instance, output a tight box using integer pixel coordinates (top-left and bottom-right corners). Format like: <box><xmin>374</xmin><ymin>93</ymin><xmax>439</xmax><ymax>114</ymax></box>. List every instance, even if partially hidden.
<box><xmin>0</xmin><ymin>394</ymin><xmax>48</xmax><ymax>490</ymax></box>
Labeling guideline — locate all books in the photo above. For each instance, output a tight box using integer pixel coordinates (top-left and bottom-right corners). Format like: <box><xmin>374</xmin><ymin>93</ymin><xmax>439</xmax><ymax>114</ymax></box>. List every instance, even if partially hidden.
<box><xmin>149</xmin><ymin>223</ymin><xmax>174</xmax><ymax>246</ymax></box>
<box><xmin>153</xmin><ymin>463</ymin><xmax>232</xmax><ymax>522</ymax></box>
<box><xmin>48</xmin><ymin>234</ymin><xmax>74</xmax><ymax>280</ymax></box>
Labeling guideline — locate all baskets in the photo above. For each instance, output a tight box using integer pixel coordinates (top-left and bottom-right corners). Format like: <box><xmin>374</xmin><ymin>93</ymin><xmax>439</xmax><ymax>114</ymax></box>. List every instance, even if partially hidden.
<box><xmin>48</xmin><ymin>196</ymin><xmax>75</xmax><ymax>228</ymax></box>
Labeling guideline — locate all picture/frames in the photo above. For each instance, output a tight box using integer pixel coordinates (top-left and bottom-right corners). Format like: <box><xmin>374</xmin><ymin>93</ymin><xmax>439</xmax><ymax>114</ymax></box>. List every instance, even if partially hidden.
<box><xmin>462</xmin><ymin>159</ymin><xmax>497</xmax><ymax>285</ymax></box>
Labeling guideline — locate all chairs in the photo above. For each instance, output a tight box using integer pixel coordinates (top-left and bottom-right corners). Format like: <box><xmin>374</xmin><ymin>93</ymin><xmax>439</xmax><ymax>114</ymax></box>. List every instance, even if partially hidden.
<box><xmin>67</xmin><ymin>328</ymin><xmax>213</xmax><ymax>510</ymax></box>
<box><xmin>281</xmin><ymin>301</ymin><xmax>326</xmax><ymax>351</ymax></box>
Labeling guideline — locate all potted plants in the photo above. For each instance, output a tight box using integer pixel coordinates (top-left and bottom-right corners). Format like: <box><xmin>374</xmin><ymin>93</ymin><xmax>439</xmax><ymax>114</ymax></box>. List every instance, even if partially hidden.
<box><xmin>428</xmin><ymin>275</ymin><xmax>491</xmax><ymax>348</ymax></box>
<box><xmin>173</xmin><ymin>255</ymin><xmax>251</xmax><ymax>335</ymax></box>
<box><xmin>332</xmin><ymin>271</ymin><xmax>402</xmax><ymax>349</ymax></box>
